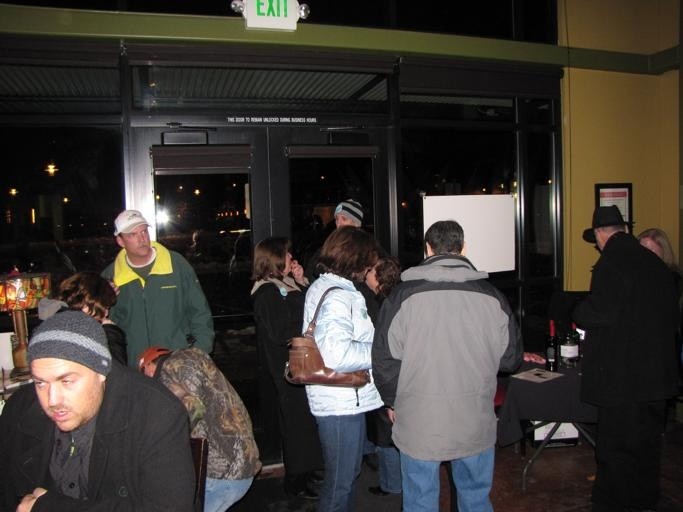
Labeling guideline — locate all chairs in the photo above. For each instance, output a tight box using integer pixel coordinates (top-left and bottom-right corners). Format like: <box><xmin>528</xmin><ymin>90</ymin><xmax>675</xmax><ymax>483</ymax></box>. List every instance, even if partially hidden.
<box><xmin>0</xmin><ymin>332</ymin><xmax>17</xmax><ymax>370</ymax></box>
<box><xmin>191</xmin><ymin>435</ymin><xmax>208</xmax><ymax>512</ymax></box>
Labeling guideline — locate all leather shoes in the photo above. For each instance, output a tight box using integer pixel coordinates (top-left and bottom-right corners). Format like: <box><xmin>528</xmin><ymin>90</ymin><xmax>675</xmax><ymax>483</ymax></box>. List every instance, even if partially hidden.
<box><xmin>307</xmin><ymin>472</ymin><xmax>325</xmax><ymax>483</ymax></box>
<box><xmin>367</xmin><ymin>483</ymin><xmax>393</xmax><ymax>495</ymax></box>
<box><xmin>286</xmin><ymin>485</ymin><xmax>318</xmax><ymax>500</ymax></box>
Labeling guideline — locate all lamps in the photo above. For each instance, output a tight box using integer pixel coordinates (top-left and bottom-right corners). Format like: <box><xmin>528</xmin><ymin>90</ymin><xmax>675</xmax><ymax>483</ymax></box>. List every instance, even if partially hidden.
<box><xmin>1</xmin><ymin>264</ymin><xmax>53</xmax><ymax>383</ymax></box>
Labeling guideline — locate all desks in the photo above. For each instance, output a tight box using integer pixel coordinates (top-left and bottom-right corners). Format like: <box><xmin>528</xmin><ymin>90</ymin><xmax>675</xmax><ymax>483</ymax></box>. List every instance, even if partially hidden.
<box><xmin>509</xmin><ymin>347</ymin><xmax>602</xmax><ymax>491</ymax></box>
<box><xmin>1</xmin><ymin>370</ymin><xmax>43</xmax><ymax>400</ymax></box>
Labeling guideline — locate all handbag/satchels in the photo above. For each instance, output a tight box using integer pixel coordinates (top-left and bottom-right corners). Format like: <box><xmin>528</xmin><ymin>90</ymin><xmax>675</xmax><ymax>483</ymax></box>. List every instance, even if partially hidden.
<box><xmin>283</xmin><ymin>337</ymin><xmax>370</xmax><ymax>387</ymax></box>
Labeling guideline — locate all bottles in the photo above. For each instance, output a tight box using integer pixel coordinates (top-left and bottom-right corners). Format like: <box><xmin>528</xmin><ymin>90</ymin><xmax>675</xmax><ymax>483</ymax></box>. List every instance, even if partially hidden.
<box><xmin>545</xmin><ymin>319</ymin><xmax>579</xmax><ymax>372</ymax></box>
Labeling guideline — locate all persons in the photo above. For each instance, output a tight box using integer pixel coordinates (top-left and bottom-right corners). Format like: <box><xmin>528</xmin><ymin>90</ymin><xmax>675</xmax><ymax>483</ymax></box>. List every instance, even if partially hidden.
<box><xmin>175</xmin><ymin>222</ymin><xmax>252</xmax><ymax>308</ymax></box>
<box><xmin>253</xmin><ymin>237</ymin><xmax>321</xmax><ymax>499</ymax></box>
<box><xmin>360</xmin><ymin>259</ymin><xmax>404</xmax><ymax>496</ymax></box>
<box><xmin>0</xmin><ymin>310</ymin><xmax>193</xmax><ymax>511</ymax></box>
<box><xmin>303</xmin><ymin>227</ymin><xmax>377</xmax><ymax>512</ymax></box>
<box><xmin>374</xmin><ymin>222</ymin><xmax>523</xmax><ymax>512</ymax></box>
<box><xmin>101</xmin><ymin>209</ymin><xmax>215</xmax><ymax>368</ymax></box>
<box><xmin>571</xmin><ymin>205</ymin><xmax>683</xmax><ymax>507</ymax></box>
<box><xmin>635</xmin><ymin>227</ymin><xmax>675</xmax><ymax>268</ymax></box>
<box><xmin>135</xmin><ymin>348</ymin><xmax>261</xmax><ymax>511</ymax></box>
<box><xmin>37</xmin><ymin>273</ymin><xmax>126</xmax><ymax>362</ymax></box>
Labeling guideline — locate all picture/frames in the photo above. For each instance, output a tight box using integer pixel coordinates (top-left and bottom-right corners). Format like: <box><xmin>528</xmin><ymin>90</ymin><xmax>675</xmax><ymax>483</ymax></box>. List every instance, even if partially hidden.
<box><xmin>595</xmin><ymin>183</ymin><xmax>634</xmax><ymax>231</ymax></box>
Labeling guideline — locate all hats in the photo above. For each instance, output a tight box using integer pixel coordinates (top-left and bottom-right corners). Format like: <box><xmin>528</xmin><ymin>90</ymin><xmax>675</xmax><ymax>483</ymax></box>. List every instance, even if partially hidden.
<box><xmin>113</xmin><ymin>210</ymin><xmax>149</xmax><ymax>236</ymax></box>
<box><xmin>136</xmin><ymin>345</ymin><xmax>171</xmax><ymax>373</ymax></box>
<box><xmin>25</xmin><ymin>310</ymin><xmax>112</xmax><ymax>376</ymax></box>
<box><xmin>582</xmin><ymin>204</ymin><xmax>634</xmax><ymax>242</ymax></box>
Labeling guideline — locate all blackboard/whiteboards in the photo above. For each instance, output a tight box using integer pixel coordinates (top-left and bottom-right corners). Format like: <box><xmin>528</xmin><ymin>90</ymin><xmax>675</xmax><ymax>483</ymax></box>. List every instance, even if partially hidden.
<box><xmin>422</xmin><ymin>194</ymin><xmax>516</xmax><ymax>274</ymax></box>
